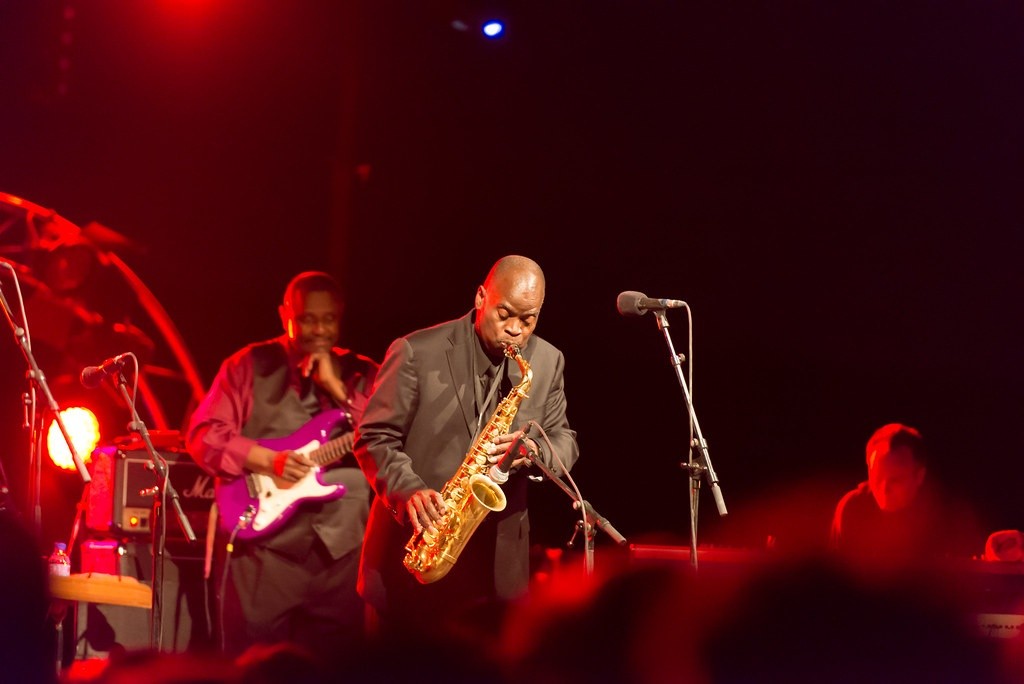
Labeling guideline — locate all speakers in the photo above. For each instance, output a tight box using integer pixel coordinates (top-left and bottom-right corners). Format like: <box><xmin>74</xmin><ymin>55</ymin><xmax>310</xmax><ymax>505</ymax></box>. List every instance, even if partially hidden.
<box><xmin>76</xmin><ymin>538</ymin><xmax>218</xmax><ymax>663</ymax></box>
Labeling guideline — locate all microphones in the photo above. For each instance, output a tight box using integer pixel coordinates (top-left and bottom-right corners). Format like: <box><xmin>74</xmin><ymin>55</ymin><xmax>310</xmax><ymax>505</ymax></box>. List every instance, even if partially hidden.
<box><xmin>80</xmin><ymin>353</ymin><xmax>131</xmax><ymax>389</ymax></box>
<box><xmin>489</xmin><ymin>422</ymin><xmax>534</xmax><ymax>484</ymax></box>
<box><xmin>617</xmin><ymin>291</ymin><xmax>685</xmax><ymax>316</ymax></box>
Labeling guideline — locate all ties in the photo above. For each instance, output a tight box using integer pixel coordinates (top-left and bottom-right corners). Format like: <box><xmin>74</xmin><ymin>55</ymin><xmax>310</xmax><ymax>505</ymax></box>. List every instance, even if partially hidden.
<box><xmin>488</xmin><ymin>363</ymin><xmax>502</xmax><ymax>424</ymax></box>
<box><xmin>300</xmin><ymin>368</ymin><xmax>322</xmax><ymax>419</ymax></box>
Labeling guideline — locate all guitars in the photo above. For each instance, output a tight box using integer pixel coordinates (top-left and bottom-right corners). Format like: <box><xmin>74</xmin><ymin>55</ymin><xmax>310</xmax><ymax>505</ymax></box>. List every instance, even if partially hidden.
<box><xmin>213</xmin><ymin>408</ymin><xmax>354</xmax><ymax>540</ymax></box>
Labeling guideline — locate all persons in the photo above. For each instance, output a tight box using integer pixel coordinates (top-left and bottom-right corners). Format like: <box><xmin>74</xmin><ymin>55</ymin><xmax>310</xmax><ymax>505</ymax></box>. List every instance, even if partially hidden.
<box><xmin>185</xmin><ymin>271</ymin><xmax>380</xmax><ymax>662</ymax></box>
<box><xmin>353</xmin><ymin>255</ymin><xmax>579</xmax><ymax>648</ymax></box>
<box><xmin>829</xmin><ymin>422</ymin><xmax>991</xmax><ymax>614</ymax></box>
<box><xmin>1</xmin><ymin>508</ymin><xmax>1024</xmax><ymax>684</ymax></box>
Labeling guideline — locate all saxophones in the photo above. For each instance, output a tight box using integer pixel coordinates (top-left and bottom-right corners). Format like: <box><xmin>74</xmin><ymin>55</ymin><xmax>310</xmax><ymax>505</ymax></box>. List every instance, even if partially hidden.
<box><xmin>399</xmin><ymin>338</ymin><xmax>535</xmax><ymax>585</ymax></box>
